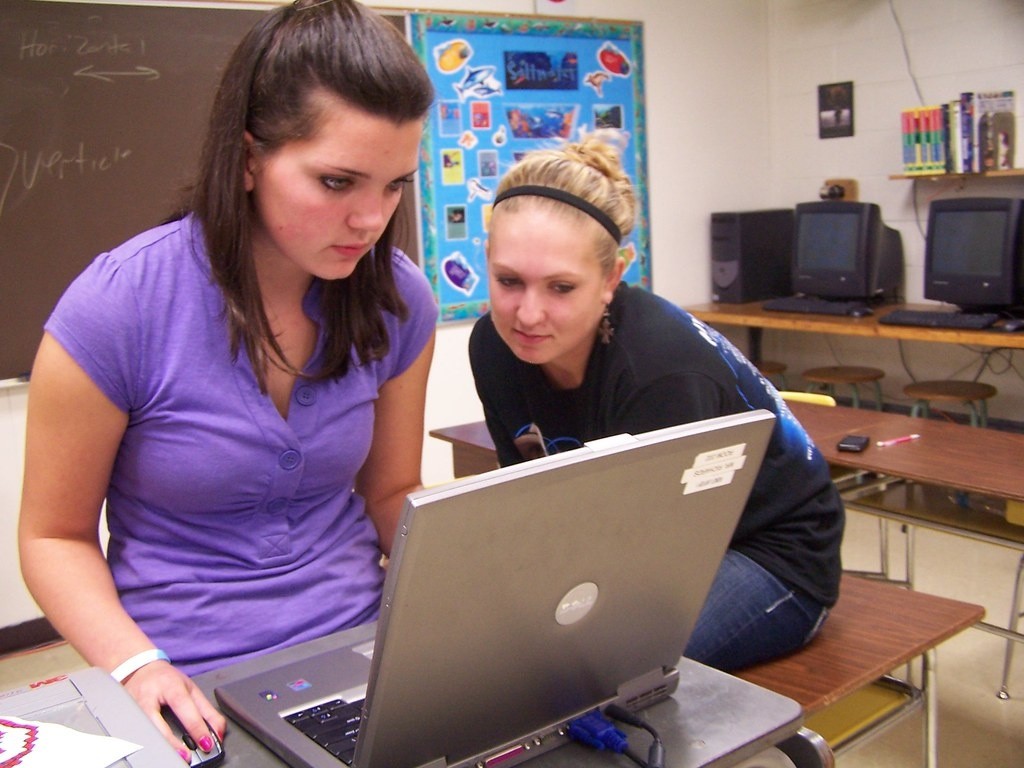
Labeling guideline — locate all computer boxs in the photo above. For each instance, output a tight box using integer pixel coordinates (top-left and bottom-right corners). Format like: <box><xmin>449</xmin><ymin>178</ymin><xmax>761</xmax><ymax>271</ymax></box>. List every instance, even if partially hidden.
<box><xmin>710</xmin><ymin>209</ymin><xmax>799</xmax><ymax>303</ymax></box>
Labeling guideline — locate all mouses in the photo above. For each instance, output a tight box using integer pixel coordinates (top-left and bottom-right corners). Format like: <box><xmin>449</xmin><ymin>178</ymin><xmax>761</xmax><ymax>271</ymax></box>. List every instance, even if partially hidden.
<box><xmin>851</xmin><ymin>306</ymin><xmax>875</xmax><ymax>317</ymax></box>
<box><xmin>1004</xmin><ymin>318</ymin><xmax>1024</xmax><ymax>332</ymax></box>
<box><xmin>160</xmin><ymin>703</ymin><xmax>225</xmax><ymax>768</ymax></box>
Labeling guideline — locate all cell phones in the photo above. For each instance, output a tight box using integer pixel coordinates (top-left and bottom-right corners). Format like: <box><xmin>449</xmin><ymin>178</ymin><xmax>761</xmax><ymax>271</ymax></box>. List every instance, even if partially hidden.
<box><xmin>838</xmin><ymin>434</ymin><xmax>869</xmax><ymax>451</ymax></box>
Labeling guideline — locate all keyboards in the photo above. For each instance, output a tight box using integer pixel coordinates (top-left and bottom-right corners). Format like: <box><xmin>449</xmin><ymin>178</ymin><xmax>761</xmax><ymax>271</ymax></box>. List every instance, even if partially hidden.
<box><xmin>763</xmin><ymin>298</ymin><xmax>863</xmax><ymax>315</ymax></box>
<box><xmin>879</xmin><ymin>309</ymin><xmax>1000</xmax><ymax>330</ymax></box>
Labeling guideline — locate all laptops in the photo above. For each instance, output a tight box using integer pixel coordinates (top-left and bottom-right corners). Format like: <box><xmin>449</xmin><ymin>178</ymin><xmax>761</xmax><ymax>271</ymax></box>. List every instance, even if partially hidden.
<box><xmin>213</xmin><ymin>407</ymin><xmax>776</xmax><ymax>768</ymax></box>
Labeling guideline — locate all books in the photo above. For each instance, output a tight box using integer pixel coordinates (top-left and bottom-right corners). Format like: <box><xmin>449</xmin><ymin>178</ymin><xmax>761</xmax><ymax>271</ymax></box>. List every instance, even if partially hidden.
<box><xmin>900</xmin><ymin>89</ymin><xmax>1015</xmax><ymax>174</ymax></box>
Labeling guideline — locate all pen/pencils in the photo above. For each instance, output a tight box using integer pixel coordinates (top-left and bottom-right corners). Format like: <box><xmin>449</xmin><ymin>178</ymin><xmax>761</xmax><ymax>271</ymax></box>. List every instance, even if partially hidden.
<box><xmin>875</xmin><ymin>434</ymin><xmax>921</xmax><ymax>447</ymax></box>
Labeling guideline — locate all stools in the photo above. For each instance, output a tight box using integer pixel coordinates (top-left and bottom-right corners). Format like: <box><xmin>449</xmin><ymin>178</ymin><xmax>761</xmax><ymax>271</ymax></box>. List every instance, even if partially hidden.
<box><xmin>801</xmin><ymin>365</ymin><xmax>885</xmax><ymax>412</ymax></box>
<box><xmin>752</xmin><ymin>360</ymin><xmax>788</xmax><ymax>391</ymax></box>
<box><xmin>903</xmin><ymin>380</ymin><xmax>996</xmax><ymax>426</ymax></box>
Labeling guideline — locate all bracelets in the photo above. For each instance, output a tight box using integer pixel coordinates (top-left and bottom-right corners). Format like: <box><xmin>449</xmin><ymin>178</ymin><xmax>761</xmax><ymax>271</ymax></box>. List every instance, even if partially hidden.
<box><xmin>109</xmin><ymin>648</ymin><xmax>171</xmax><ymax>683</ymax></box>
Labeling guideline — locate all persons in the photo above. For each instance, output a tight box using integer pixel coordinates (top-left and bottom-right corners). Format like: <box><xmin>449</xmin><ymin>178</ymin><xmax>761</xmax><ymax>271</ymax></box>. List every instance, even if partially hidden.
<box><xmin>819</xmin><ymin>87</ymin><xmax>850</xmax><ymax>126</ymax></box>
<box><xmin>469</xmin><ymin>130</ymin><xmax>845</xmax><ymax>672</ymax></box>
<box><xmin>17</xmin><ymin>0</ymin><xmax>438</xmax><ymax>764</ymax></box>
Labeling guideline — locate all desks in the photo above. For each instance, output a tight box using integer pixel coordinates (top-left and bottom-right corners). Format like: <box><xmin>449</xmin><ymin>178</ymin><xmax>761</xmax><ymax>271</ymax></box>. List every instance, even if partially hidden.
<box><xmin>141</xmin><ymin>397</ymin><xmax>1024</xmax><ymax>768</ymax></box>
<box><xmin>683</xmin><ymin>304</ymin><xmax>1024</xmax><ymax>366</ymax></box>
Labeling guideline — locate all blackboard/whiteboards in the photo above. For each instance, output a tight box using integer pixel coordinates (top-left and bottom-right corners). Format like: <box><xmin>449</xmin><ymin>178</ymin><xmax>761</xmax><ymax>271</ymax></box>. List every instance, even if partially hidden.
<box><xmin>0</xmin><ymin>0</ymin><xmax>424</xmax><ymax>382</ymax></box>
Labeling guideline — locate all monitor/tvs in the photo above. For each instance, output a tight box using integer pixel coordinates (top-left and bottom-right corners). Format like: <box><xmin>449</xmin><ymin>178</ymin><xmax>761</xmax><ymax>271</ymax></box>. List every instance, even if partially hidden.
<box><xmin>922</xmin><ymin>197</ymin><xmax>1024</xmax><ymax>319</ymax></box>
<box><xmin>794</xmin><ymin>202</ymin><xmax>903</xmax><ymax>301</ymax></box>
